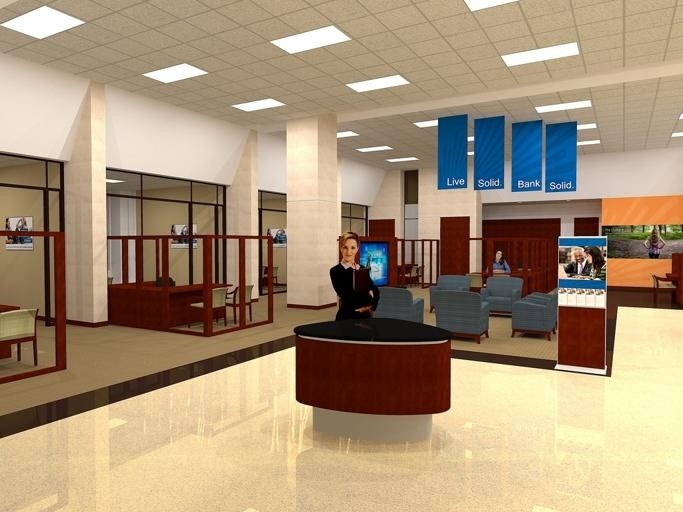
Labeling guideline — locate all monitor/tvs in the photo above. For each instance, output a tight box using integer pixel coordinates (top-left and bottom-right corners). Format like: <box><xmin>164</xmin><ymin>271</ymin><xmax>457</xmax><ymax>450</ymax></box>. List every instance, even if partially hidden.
<box><xmin>358</xmin><ymin>241</ymin><xmax>390</xmax><ymax>286</ymax></box>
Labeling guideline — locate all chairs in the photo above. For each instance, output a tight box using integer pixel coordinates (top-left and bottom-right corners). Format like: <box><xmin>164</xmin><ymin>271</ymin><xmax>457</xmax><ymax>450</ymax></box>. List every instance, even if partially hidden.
<box><xmin>188</xmin><ymin>283</ymin><xmax>253</xmax><ymax>327</ymax></box>
<box><xmin>372</xmin><ymin>275</ymin><xmax>558</xmax><ymax>345</ymax></box>
<box><xmin>264</xmin><ymin>266</ymin><xmax>278</xmax><ymax>285</ymax></box>
<box><xmin>0</xmin><ymin>307</ymin><xmax>39</xmax><ymax>367</ymax></box>
<box><xmin>399</xmin><ymin>264</ymin><xmax>424</xmax><ymax>287</ymax></box>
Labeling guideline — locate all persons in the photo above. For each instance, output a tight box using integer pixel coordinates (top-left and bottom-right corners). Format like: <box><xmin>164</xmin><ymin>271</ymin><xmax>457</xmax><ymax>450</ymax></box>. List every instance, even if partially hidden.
<box><xmin>570</xmin><ymin>244</ymin><xmax>605</xmax><ymax>281</ymax></box>
<box><xmin>329</xmin><ymin>231</ymin><xmax>381</xmax><ymax>320</ymax></box>
<box><xmin>266</xmin><ymin>229</ymin><xmax>271</xmax><ymax>243</ymax></box>
<box><xmin>485</xmin><ymin>247</ymin><xmax>510</xmax><ymax>272</ymax></box>
<box><xmin>170</xmin><ymin>224</ymin><xmax>177</xmax><ymax>244</ymax></box>
<box><xmin>642</xmin><ymin>227</ymin><xmax>666</xmax><ymax>258</ymax></box>
<box><xmin>564</xmin><ymin>246</ymin><xmax>588</xmax><ymax>274</ymax></box>
<box><xmin>4</xmin><ymin>218</ymin><xmax>13</xmax><ymax>244</ymax></box>
